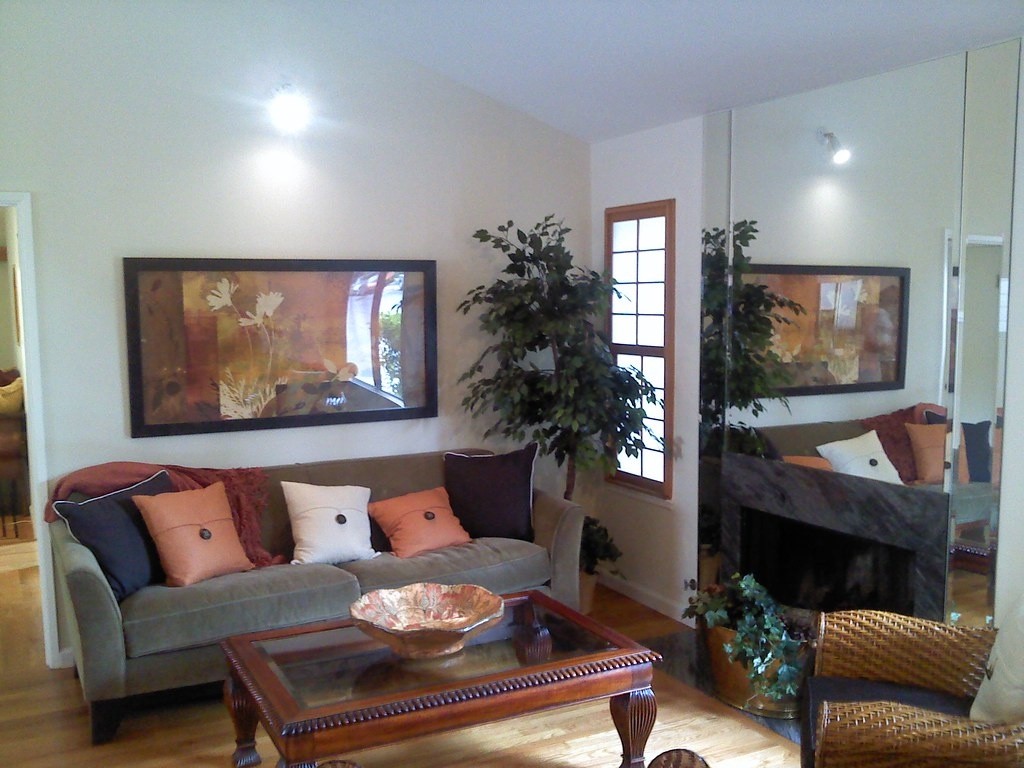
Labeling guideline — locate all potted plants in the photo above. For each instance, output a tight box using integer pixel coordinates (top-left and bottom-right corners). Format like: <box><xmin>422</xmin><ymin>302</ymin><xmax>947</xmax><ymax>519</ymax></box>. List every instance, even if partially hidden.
<box><xmin>456</xmin><ymin>213</ymin><xmax>674</xmax><ymax>616</ymax></box>
<box><xmin>681</xmin><ymin>570</ymin><xmax>822</xmax><ymax>720</ymax></box>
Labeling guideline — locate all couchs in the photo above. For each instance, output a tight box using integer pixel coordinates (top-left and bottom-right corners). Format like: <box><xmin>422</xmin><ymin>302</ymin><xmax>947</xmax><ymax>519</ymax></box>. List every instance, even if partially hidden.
<box><xmin>43</xmin><ymin>440</ymin><xmax>586</xmax><ymax>749</ymax></box>
<box><xmin>702</xmin><ymin>401</ymin><xmax>1004</xmax><ymax>557</ymax></box>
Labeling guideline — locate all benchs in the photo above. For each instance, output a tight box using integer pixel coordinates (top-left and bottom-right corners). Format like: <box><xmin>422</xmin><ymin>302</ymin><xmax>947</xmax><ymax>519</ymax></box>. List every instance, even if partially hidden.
<box><xmin>0</xmin><ymin>368</ymin><xmax>30</xmax><ymax>537</ymax></box>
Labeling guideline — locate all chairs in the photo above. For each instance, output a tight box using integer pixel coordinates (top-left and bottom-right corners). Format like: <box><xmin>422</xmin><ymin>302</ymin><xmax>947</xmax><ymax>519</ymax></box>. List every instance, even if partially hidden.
<box><xmin>797</xmin><ymin>588</ymin><xmax>1024</xmax><ymax>768</ymax></box>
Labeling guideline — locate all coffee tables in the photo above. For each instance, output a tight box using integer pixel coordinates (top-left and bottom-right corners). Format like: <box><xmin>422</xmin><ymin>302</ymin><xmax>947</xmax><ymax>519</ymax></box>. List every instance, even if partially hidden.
<box><xmin>216</xmin><ymin>589</ymin><xmax>664</xmax><ymax>768</ymax></box>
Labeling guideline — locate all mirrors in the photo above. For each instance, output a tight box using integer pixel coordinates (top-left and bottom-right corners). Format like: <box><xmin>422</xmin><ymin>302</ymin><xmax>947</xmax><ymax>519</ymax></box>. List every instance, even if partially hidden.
<box><xmin>695</xmin><ymin>31</ymin><xmax>1021</xmax><ymax>630</ymax></box>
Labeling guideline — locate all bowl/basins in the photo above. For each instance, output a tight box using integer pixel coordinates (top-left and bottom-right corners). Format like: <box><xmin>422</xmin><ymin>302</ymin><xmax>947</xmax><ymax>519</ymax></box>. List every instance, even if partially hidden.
<box><xmin>349</xmin><ymin>583</ymin><xmax>504</xmax><ymax>658</ymax></box>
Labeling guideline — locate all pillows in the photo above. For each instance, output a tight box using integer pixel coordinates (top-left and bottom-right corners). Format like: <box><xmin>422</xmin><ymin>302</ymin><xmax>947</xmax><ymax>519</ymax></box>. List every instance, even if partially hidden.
<box><xmin>279</xmin><ymin>480</ymin><xmax>382</xmax><ymax>565</ymax></box>
<box><xmin>782</xmin><ymin>456</ymin><xmax>834</xmax><ymax>472</ymax></box>
<box><xmin>52</xmin><ymin>470</ymin><xmax>175</xmax><ymax>605</ymax></box>
<box><xmin>131</xmin><ymin>481</ymin><xmax>256</xmax><ymax>587</ymax></box>
<box><xmin>442</xmin><ymin>441</ymin><xmax>539</xmax><ymax>543</ymax></box>
<box><xmin>368</xmin><ymin>486</ymin><xmax>475</xmax><ymax>559</ymax></box>
<box><xmin>814</xmin><ymin>430</ymin><xmax>905</xmax><ymax>485</ymax></box>
<box><xmin>904</xmin><ymin>423</ymin><xmax>973</xmax><ymax>486</ymax></box>
<box><xmin>0</xmin><ymin>376</ymin><xmax>23</xmax><ymax>414</ymax></box>
<box><xmin>922</xmin><ymin>409</ymin><xmax>992</xmax><ymax>483</ymax></box>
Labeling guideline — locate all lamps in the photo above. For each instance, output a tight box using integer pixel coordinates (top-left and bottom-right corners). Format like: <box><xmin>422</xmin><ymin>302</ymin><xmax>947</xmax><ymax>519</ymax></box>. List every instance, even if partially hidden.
<box><xmin>817</xmin><ymin>128</ymin><xmax>851</xmax><ymax>166</ymax></box>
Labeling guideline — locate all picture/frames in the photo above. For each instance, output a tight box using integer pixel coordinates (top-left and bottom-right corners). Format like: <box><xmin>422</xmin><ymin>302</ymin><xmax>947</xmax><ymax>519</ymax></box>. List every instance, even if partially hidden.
<box><xmin>123</xmin><ymin>256</ymin><xmax>438</xmax><ymax>439</ymax></box>
<box><xmin>700</xmin><ymin>262</ymin><xmax>911</xmax><ymax>399</ymax></box>
